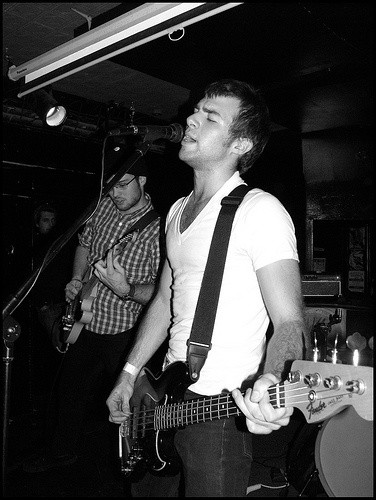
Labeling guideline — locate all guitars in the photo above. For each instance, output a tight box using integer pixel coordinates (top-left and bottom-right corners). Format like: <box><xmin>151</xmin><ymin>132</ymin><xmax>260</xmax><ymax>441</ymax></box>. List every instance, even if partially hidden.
<box><xmin>114</xmin><ymin>343</ymin><xmax>375</xmax><ymax>481</ymax></box>
<box><xmin>56</xmin><ymin>230</ymin><xmax>139</xmax><ymax>344</ymax></box>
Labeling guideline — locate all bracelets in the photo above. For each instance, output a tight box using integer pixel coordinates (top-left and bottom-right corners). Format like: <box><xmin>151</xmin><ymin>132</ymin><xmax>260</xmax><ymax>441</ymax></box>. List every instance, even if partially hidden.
<box><xmin>123</xmin><ymin>361</ymin><xmax>140</xmax><ymax>377</ymax></box>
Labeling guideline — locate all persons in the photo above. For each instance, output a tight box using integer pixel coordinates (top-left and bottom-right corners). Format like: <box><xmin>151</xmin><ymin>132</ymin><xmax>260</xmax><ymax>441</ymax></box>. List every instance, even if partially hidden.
<box><xmin>107</xmin><ymin>77</ymin><xmax>311</xmax><ymax>500</ymax></box>
<box><xmin>25</xmin><ymin>199</ymin><xmax>59</xmax><ymax>247</ymax></box>
<box><xmin>64</xmin><ymin>144</ymin><xmax>161</xmax><ymax>368</ymax></box>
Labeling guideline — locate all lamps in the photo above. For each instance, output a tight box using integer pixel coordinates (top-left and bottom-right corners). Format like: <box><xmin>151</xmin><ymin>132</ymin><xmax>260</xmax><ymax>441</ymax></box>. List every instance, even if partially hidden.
<box><xmin>24</xmin><ymin>88</ymin><xmax>68</xmax><ymax>127</ymax></box>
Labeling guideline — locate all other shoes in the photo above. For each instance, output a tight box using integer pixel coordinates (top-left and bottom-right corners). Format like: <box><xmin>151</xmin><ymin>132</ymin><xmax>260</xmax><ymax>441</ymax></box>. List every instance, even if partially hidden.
<box><xmin>249</xmin><ymin>469</ymin><xmax>290</xmax><ymax>489</ymax></box>
<box><xmin>22</xmin><ymin>445</ymin><xmax>81</xmax><ymax>472</ymax></box>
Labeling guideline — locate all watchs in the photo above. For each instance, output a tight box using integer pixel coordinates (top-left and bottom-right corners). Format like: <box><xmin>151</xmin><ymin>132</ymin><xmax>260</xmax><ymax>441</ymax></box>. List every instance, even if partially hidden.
<box><xmin>119</xmin><ymin>283</ymin><xmax>135</xmax><ymax>301</ymax></box>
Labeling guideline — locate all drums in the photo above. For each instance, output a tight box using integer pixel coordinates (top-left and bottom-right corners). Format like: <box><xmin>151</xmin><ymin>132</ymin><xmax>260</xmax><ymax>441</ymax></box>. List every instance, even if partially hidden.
<box><xmin>314</xmin><ymin>405</ymin><xmax>374</xmax><ymax>498</ymax></box>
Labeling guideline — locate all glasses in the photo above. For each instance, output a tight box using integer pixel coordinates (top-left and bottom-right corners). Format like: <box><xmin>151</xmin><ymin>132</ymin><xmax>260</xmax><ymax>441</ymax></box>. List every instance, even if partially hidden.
<box><xmin>42</xmin><ymin>218</ymin><xmax>56</xmax><ymax>222</ymax></box>
<box><xmin>108</xmin><ymin>176</ymin><xmax>136</xmax><ymax>191</ymax></box>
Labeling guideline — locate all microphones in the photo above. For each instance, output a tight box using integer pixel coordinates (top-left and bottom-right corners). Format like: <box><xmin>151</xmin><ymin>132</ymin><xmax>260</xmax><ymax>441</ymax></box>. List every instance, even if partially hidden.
<box><xmin>108</xmin><ymin>123</ymin><xmax>183</xmax><ymax>143</ymax></box>
